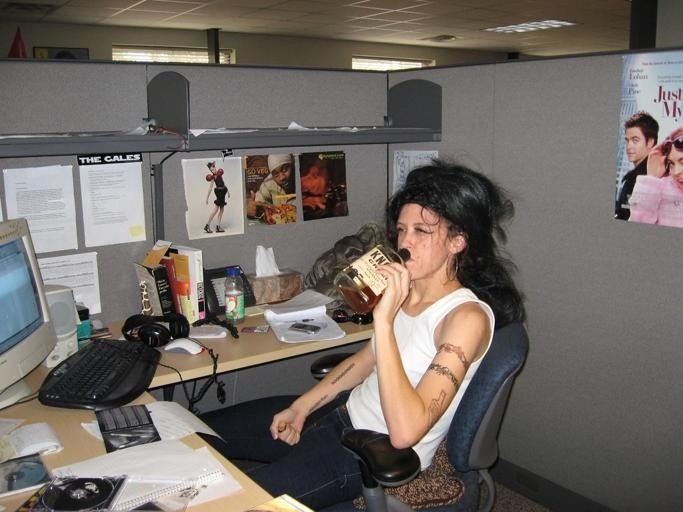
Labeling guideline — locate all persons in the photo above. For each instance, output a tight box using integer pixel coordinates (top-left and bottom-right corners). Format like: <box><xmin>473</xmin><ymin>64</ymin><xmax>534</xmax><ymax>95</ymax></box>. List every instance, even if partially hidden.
<box><xmin>204</xmin><ymin>162</ymin><xmax>229</xmax><ymax>234</ymax></box>
<box><xmin>300</xmin><ymin>157</ymin><xmax>332</xmax><ymax>209</ymax></box>
<box><xmin>616</xmin><ymin>113</ymin><xmax>660</xmax><ymax>219</ymax></box>
<box><xmin>193</xmin><ymin>159</ymin><xmax>528</xmax><ymax>512</ymax></box>
<box><xmin>628</xmin><ymin>130</ymin><xmax>683</xmax><ymax>227</ymax></box>
<box><xmin>254</xmin><ymin>155</ymin><xmax>295</xmax><ymax>223</ymax></box>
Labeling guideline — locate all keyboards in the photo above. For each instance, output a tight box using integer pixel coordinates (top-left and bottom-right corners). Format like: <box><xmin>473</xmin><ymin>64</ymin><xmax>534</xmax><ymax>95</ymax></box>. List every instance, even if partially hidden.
<box><xmin>39</xmin><ymin>338</ymin><xmax>162</xmax><ymax>412</ymax></box>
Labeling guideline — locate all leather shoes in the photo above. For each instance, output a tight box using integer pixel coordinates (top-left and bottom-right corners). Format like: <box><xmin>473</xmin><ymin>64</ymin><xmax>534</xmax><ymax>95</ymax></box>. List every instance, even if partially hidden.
<box><xmin>216</xmin><ymin>225</ymin><xmax>226</xmax><ymax>233</ymax></box>
<box><xmin>204</xmin><ymin>225</ymin><xmax>214</xmax><ymax>234</ymax></box>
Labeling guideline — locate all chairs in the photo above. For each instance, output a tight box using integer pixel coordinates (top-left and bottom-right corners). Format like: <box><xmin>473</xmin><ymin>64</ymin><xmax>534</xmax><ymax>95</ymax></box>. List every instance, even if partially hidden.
<box><xmin>223</xmin><ymin>321</ymin><xmax>530</xmax><ymax>512</ymax></box>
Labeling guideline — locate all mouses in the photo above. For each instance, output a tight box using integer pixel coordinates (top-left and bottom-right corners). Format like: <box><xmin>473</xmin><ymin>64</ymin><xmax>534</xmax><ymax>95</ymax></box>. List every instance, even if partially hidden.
<box><xmin>165</xmin><ymin>338</ymin><xmax>205</xmax><ymax>355</ymax></box>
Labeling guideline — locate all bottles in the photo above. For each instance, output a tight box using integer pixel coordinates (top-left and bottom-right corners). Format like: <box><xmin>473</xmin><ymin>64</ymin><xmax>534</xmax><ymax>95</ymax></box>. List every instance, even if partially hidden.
<box><xmin>332</xmin><ymin>243</ymin><xmax>411</xmax><ymax>316</ymax></box>
<box><xmin>224</xmin><ymin>267</ymin><xmax>245</xmax><ymax>326</ymax></box>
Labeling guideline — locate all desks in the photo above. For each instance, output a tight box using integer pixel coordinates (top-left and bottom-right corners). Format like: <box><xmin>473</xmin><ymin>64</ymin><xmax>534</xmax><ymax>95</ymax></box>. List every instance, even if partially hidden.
<box><xmin>0</xmin><ymin>291</ymin><xmax>374</xmax><ymax>512</ymax></box>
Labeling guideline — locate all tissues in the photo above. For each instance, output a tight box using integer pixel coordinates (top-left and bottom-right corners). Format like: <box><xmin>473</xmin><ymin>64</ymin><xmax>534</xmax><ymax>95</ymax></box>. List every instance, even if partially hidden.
<box><xmin>245</xmin><ymin>246</ymin><xmax>304</xmax><ymax>305</ymax></box>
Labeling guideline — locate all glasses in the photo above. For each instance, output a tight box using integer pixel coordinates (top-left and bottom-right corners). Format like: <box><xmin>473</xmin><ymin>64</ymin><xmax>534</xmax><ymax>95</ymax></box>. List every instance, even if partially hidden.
<box><xmin>662</xmin><ymin>137</ymin><xmax>683</xmax><ymax>153</ymax></box>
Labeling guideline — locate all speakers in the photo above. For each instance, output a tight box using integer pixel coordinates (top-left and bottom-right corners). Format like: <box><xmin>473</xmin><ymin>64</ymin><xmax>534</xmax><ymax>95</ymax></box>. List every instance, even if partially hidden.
<box><xmin>42</xmin><ymin>284</ymin><xmax>79</xmax><ymax>367</ymax></box>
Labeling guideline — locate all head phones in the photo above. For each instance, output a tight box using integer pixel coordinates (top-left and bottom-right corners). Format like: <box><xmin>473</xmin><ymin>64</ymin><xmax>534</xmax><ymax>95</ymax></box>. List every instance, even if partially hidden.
<box><xmin>121</xmin><ymin>313</ymin><xmax>190</xmax><ymax>347</ymax></box>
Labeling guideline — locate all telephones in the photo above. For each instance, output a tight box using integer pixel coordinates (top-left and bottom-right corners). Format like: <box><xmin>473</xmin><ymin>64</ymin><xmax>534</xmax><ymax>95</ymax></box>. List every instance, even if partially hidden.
<box><xmin>203</xmin><ymin>265</ymin><xmax>256</xmax><ymax>316</ymax></box>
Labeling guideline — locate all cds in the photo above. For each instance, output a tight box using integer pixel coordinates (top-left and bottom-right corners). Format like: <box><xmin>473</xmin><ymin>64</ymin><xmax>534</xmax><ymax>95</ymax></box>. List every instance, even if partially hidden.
<box><xmin>1</xmin><ymin>462</ymin><xmax>46</xmax><ymax>492</ymax></box>
<box><xmin>42</xmin><ymin>478</ymin><xmax>115</xmax><ymax>512</ymax></box>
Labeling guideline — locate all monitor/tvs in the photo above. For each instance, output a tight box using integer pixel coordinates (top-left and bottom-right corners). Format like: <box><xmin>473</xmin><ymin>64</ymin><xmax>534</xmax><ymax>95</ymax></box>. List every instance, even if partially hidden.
<box><xmin>1</xmin><ymin>218</ymin><xmax>58</xmax><ymax>409</ymax></box>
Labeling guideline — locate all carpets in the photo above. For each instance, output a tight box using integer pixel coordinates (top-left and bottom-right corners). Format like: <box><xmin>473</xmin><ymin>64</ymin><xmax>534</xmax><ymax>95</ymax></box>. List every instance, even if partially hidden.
<box><xmin>478</xmin><ymin>475</ymin><xmax>553</xmax><ymax>512</ymax></box>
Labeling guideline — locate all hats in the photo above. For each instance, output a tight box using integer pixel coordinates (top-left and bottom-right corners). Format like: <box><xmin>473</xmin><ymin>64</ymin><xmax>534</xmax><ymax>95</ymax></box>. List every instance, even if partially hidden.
<box><xmin>207</xmin><ymin>161</ymin><xmax>216</xmax><ymax>167</ymax></box>
<box><xmin>268</xmin><ymin>154</ymin><xmax>294</xmax><ymax>172</ymax></box>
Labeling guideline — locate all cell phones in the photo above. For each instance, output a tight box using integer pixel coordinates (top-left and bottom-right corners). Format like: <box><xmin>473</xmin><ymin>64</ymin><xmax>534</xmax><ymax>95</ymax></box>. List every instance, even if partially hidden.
<box><xmin>288</xmin><ymin>323</ymin><xmax>321</xmax><ymax>335</ymax></box>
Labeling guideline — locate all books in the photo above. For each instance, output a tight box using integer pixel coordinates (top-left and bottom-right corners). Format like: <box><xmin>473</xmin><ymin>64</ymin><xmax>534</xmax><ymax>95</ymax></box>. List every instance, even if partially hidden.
<box><xmin>52</xmin><ymin>439</ymin><xmax>222</xmax><ymax>512</ymax></box>
<box><xmin>132</xmin><ymin>242</ymin><xmax>207</xmax><ymax>325</ymax></box>
